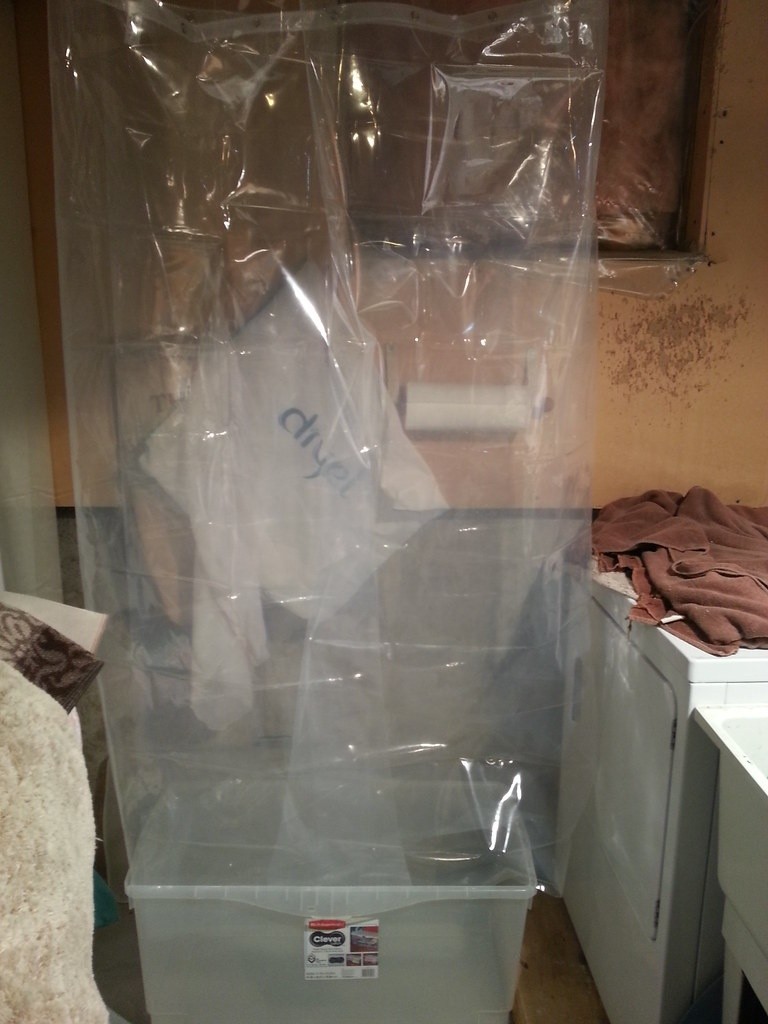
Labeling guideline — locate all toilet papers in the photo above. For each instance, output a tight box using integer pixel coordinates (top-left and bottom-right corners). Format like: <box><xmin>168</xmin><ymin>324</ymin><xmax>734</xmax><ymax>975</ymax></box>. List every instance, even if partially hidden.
<box><xmin>402</xmin><ymin>380</ymin><xmax>531</xmax><ymax>430</ymax></box>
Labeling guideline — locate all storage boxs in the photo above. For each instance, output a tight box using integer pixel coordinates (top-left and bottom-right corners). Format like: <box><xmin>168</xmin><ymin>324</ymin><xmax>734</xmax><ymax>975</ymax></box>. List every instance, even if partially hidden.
<box><xmin>125</xmin><ymin>776</ymin><xmax>538</xmax><ymax>1024</ymax></box>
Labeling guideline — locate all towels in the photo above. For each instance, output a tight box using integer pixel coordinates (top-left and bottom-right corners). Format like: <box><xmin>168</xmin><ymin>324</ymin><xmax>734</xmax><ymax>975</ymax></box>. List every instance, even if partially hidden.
<box><xmin>134</xmin><ymin>260</ymin><xmax>455</xmax><ymax>730</ymax></box>
<box><xmin>558</xmin><ymin>483</ymin><xmax>767</xmax><ymax>659</ymax></box>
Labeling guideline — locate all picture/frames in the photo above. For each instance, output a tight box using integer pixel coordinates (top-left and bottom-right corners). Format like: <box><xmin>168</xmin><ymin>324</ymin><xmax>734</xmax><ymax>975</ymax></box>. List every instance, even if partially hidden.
<box><xmin>335</xmin><ymin>0</ymin><xmax>719</xmax><ymax>263</ymax></box>
